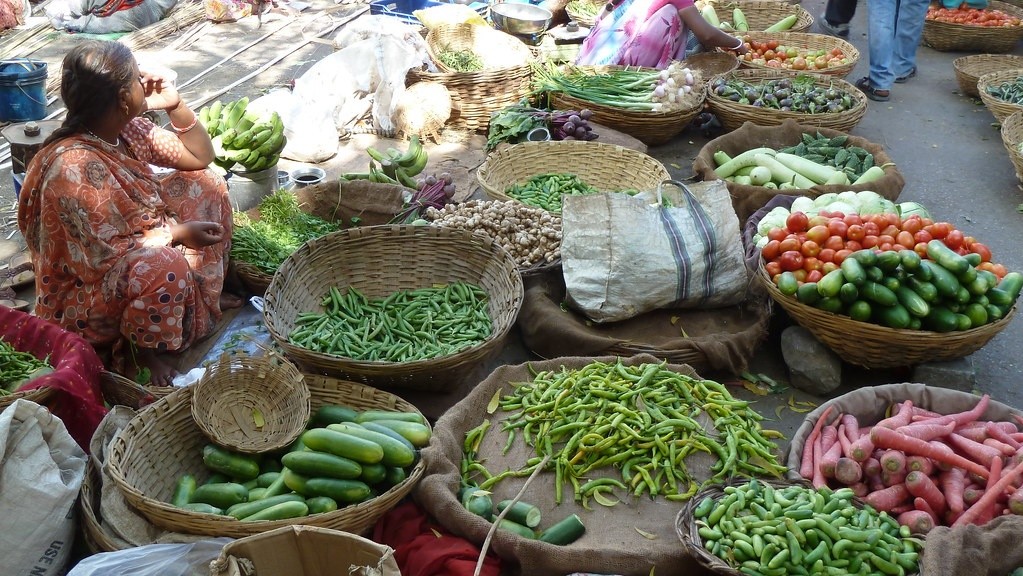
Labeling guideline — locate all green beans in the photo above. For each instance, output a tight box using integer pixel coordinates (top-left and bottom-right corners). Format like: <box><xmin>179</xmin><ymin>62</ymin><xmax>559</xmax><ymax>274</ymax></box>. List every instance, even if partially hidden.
<box><xmin>986</xmin><ymin>75</ymin><xmax>1023</xmax><ymax>106</ymax></box>
<box><xmin>0</xmin><ymin>334</ymin><xmax>54</xmax><ymax>396</ymax></box>
<box><xmin>287</xmin><ymin>279</ymin><xmax>494</xmax><ymax>362</ymax></box>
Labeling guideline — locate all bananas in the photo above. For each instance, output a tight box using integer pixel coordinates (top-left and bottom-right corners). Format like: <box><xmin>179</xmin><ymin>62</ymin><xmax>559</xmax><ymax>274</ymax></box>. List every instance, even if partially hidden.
<box><xmin>199</xmin><ymin>95</ymin><xmax>286</xmax><ymax>173</ymax></box>
<box><xmin>340</xmin><ymin>134</ymin><xmax>428</xmax><ymax>189</ymax></box>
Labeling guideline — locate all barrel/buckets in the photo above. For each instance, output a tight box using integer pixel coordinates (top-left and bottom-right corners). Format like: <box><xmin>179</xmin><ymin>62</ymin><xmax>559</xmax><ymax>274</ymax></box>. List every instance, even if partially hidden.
<box><xmin>0</xmin><ymin>57</ymin><xmax>50</xmax><ymax>125</ymax></box>
<box><xmin>207</xmin><ymin>156</ymin><xmax>280</xmax><ymax>219</ymax></box>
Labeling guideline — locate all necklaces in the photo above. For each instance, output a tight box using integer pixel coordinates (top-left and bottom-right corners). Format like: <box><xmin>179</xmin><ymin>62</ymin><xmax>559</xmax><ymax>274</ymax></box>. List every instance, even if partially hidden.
<box><xmin>82</xmin><ymin>124</ymin><xmax>121</xmax><ymax>148</ymax></box>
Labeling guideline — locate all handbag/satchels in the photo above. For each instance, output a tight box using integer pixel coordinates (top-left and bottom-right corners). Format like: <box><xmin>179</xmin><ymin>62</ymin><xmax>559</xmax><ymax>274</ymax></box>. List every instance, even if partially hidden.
<box><xmin>560</xmin><ymin>179</ymin><xmax>749</xmax><ymax>324</ymax></box>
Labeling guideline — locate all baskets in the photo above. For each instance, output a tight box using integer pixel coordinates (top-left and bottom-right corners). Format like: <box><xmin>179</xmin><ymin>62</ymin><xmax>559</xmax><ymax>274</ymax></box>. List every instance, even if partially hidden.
<box><xmin>704</xmin><ymin>68</ymin><xmax>869</xmax><ymax>134</ymax></box>
<box><xmin>713</xmin><ymin>30</ymin><xmax>861</xmax><ymax>81</ymax></box>
<box><xmin>1</xmin><ymin>304</ymin><xmax>63</xmax><ymax>413</ymax></box>
<box><xmin>391</xmin><ymin>80</ymin><xmax>452</xmax><ymax>145</ymax></box>
<box><xmin>682</xmin><ymin>51</ymin><xmax>742</xmax><ymax>82</ymax></box>
<box><xmin>80</xmin><ymin>335</ymin><xmax>434</xmax><ymax>557</ymax></box>
<box><xmin>259</xmin><ymin>225</ymin><xmax>525</xmax><ymax>394</ymax></box>
<box><xmin>475</xmin><ymin>139</ymin><xmax>671</xmax><ymax>211</ymax></box>
<box><xmin>565</xmin><ymin>0</ymin><xmax>610</xmax><ymax>29</ymax></box>
<box><xmin>955</xmin><ymin>52</ymin><xmax>1023</xmax><ymax>180</ymax></box>
<box><xmin>672</xmin><ymin>476</ymin><xmax>924</xmax><ymax>576</ymax></box>
<box><xmin>694</xmin><ymin>0</ymin><xmax>816</xmax><ymax>34</ymax></box>
<box><xmin>922</xmin><ymin>0</ymin><xmax>1023</xmax><ymax>54</ymax></box>
<box><xmin>230</xmin><ymin>177</ymin><xmax>453</xmax><ymax>295</ymax></box>
<box><xmin>755</xmin><ymin>236</ymin><xmax>1014</xmax><ymax>373</ymax></box>
<box><xmin>405</xmin><ymin>23</ymin><xmax>543</xmax><ymax>135</ymax></box>
<box><xmin>418</xmin><ymin>204</ymin><xmax>565</xmax><ymax>279</ymax></box>
<box><xmin>549</xmin><ymin>65</ymin><xmax>708</xmax><ymax>146</ymax></box>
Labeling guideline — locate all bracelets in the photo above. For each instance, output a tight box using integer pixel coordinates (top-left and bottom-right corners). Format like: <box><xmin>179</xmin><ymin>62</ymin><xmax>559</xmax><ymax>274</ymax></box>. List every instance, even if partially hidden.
<box><xmin>727</xmin><ymin>39</ymin><xmax>743</xmax><ymax>51</ymax></box>
<box><xmin>168</xmin><ymin>108</ymin><xmax>198</xmax><ymax>134</ymax></box>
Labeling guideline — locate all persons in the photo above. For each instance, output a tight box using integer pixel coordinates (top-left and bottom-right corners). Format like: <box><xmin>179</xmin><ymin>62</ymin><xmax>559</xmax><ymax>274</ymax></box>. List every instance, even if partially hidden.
<box><xmin>12</xmin><ymin>39</ymin><xmax>236</xmax><ymax>358</ymax></box>
<box><xmin>816</xmin><ymin>0</ymin><xmax>858</xmax><ymax>41</ymax></box>
<box><xmin>577</xmin><ymin>0</ymin><xmax>750</xmax><ymax>80</ymax></box>
<box><xmin>856</xmin><ymin>0</ymin><xmax>930</xmax><ymax>107</ymax></box>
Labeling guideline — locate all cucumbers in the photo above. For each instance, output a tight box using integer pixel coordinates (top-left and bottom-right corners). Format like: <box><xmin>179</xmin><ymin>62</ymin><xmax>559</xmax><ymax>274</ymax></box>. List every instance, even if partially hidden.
<box><xmin>172</xmin><ymin>404</ymin><xmax>432</xmax><ymax>522</ymax></box>
<box><xmin>462</xmin><ymin>486</ymin><xmax>587</xmax><ymax>546</ymax></box>
<box><xmin>779</xmin><ymin>240</ymin><xmax>1023</xmax><ymax>333</ymax></box>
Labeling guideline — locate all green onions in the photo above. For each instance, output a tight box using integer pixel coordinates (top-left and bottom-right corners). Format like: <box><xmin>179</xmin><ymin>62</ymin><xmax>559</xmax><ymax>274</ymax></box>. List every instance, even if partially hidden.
<box><xmin>517</xmin><ymin>59</ymin><xmax>706</xmax><ymax>115</ymax></box>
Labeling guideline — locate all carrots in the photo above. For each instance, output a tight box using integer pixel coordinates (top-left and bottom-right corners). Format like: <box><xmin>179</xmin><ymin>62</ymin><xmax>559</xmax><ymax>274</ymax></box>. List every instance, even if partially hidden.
<box><xmin>799</xmin><ymin>394</ymin><xmax>1023</xmax><ymax>535</ymax></box>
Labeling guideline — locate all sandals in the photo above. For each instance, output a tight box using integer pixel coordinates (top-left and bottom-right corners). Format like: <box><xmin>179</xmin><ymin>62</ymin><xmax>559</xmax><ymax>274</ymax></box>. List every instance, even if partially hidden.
<box><xmin>854</xmin><ymin>75</ymin><xmax>889</xmax><ymax>101</ymax></box>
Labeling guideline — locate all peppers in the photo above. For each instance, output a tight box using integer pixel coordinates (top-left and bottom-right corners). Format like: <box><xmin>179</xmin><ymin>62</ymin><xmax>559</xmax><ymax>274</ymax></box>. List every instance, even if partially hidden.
<box><xmin>460</xmin><ymin>357</ymin><xmax>789</xmax><ymax>540</ymax></box>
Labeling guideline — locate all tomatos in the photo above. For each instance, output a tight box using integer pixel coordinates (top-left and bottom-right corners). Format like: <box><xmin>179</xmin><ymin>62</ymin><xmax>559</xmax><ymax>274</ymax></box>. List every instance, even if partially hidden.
<box><xmin>924</xmin><ymin>3</ymin><xmax>1023</xmax><ymax>29</ymax></box>
<box><xmin>719</xmin><ymin>35</ymin><xmax>847</xmax><ymax>70</ymax></box>
<box><xmin>763</xmin><ymin>210</ymin><xmax>1008</xmax><ymax>286</ymax></box>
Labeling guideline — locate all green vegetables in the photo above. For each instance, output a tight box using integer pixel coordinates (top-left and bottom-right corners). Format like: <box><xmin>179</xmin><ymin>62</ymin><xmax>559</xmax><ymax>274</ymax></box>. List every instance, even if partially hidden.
<box><xmin>230</xmin><ymin>188</ymin><xmax>344</xmax><ymax>277</ymax></box>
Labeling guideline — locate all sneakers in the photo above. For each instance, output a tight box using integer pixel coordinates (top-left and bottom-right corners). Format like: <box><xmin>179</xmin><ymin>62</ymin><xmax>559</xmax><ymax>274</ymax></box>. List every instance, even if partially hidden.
<box><xmin>817</xmin><ymin>9</ymin><xmax>851</xmax><ymax>39</ymax></box>
